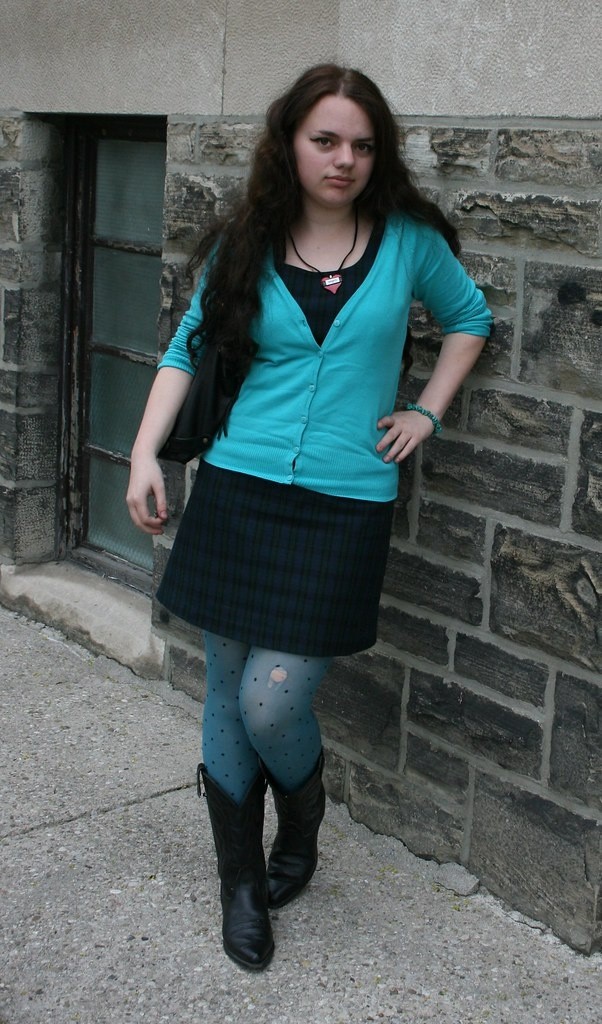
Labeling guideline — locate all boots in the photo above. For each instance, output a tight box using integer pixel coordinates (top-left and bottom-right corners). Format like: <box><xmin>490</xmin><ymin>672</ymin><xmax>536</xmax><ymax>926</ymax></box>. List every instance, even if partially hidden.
<box><xmin>197</xmin><ymin>764</ymin><xmax>274</xmax><ymax>972</ymax></box>
<box><xmin>259</xmin><ymin>744</ymin><xmax>325</xmax><ymax>910</ymax></box>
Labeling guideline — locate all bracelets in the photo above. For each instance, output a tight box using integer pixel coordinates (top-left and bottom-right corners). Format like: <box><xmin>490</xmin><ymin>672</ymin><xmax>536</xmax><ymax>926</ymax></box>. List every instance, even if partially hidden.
<box><xmin>406</xmin><ymin>403</ymin><xmax>443</xmax><ymax>436</ymax></box>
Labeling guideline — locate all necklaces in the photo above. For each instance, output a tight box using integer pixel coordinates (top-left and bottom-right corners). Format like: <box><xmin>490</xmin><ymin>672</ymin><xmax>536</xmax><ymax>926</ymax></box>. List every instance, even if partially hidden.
<box><xmin>285</xmin><ymin>205</ymin><xmax>358</xmax><ymax>294</ymax></box>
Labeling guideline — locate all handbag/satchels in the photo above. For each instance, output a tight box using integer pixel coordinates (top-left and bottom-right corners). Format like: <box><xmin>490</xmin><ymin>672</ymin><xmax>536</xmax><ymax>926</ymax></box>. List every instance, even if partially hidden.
<box><xmin>156</xmin><ymin>323</ymin><xmax>260</xmax><ymax>463</ymax></box>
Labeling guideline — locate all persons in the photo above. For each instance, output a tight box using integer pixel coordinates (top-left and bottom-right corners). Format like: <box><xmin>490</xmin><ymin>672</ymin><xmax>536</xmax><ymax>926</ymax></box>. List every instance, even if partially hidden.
<box><xmin>126</xmin><ymin>63</ymin><xmax>494</xmax><ymax>969</ymax></box>
